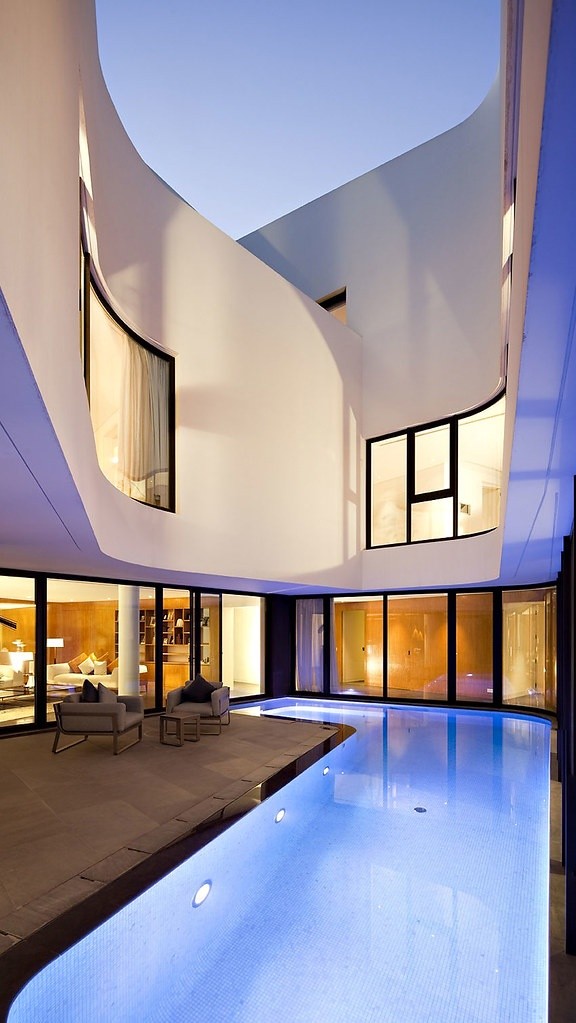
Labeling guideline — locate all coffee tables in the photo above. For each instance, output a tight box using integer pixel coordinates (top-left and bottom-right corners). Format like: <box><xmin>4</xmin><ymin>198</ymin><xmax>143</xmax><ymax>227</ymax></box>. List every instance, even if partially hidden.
<box><xmin>0</xmin><ymin>685</ymin><xmax>81</xmax><ymax>702</ymax></box>
<box><xmin>161</xmin><ymin>710</ymin><xmax>202</xmax><ymax>747</ymax></box>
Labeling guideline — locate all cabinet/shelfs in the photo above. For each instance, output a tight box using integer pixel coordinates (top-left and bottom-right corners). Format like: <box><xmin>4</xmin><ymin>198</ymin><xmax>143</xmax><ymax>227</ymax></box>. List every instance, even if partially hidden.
<box><xmin>115</xmin><ymin>609</ymin><xmax>210</xmax><ymax>663</ymax></box>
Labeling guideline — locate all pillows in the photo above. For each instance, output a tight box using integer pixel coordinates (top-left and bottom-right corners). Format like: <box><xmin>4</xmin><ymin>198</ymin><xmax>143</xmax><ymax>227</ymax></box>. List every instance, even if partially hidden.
<box><xmin>93</xmin><ymin>661</ymin><xmax>107</xmax><ymax>676</ymax></box>
<box><xmin>78</xmin><ymin>658</ymin><xmax>95</xmax><ymax>675</ymax></box>
<box><xmin>183</xmin><ymin>675</ymin><xmax>216</xmax><ymax>704</ymax></box>
<box><xmin>98</xmin><ymin>652</ymin><xmax>112</xmax><ymax>665</ymax></box>
<box><xmin>81</xmin><ymin>678</ymin><xmax>97</xmax><ymax>703</ymax></box>
<box><xmin>89</xmin><ymin>652</ymin><xmax>97</xmax><ymax>663</ymax></box>
<box><xmin>69</xmin><ymin>653</ymin><xmax>88</xmax><ymax>674</ymax></box>
<box><xmin>95</xmin><ymin>682</ymin><xmax>117</xmax><ymax>703</ymax></box>
<box><xmin>108</xmin><ymin>657</ymin><xmax>118</xmax><ymax>674</ymax></box>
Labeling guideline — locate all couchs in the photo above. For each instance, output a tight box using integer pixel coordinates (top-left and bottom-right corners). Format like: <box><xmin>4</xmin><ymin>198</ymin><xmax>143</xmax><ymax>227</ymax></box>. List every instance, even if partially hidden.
<box><xmin>165</xmin><ymin>681</ymin><xmax>230</xmax><ymax>735</ymax></box>
<box><xmin>0</xmin><ymin>664</ymin><xmax>14</xmax><ymax>689</ymax></box>
<box><xmin>47</xmin><ymin>662</ymin><xmax>148</xmax><ymax>693</ymax></box>
<box><xmin>52</xmin><ymin>692</ymin><xmax>145</xmax><ymax>754</ymax></box>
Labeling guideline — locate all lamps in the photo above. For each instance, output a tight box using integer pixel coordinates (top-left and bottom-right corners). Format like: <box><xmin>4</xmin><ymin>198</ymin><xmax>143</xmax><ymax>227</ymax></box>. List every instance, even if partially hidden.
<box><xmin>47</xmin><ymin>638</ymin><xmax>64</xmax><ymax>664</ymax></box>
